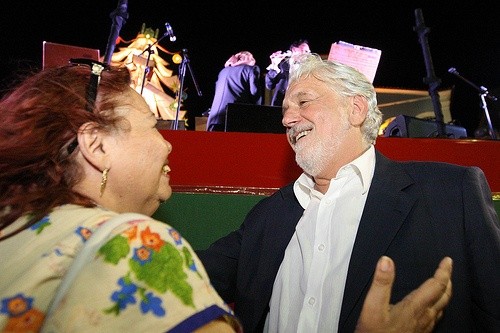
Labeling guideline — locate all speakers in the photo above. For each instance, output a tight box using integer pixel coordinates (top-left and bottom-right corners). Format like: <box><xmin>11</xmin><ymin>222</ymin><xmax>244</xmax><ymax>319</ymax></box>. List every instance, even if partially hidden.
<box><xmin>382</xmin><ymin>113</ymin><xmax>468</xmax><ymax>139</ymax></box>
<box><xmin>224</xmin><ymin>102</ymin><xmax>287</xmax><ymax>134</ymax></box>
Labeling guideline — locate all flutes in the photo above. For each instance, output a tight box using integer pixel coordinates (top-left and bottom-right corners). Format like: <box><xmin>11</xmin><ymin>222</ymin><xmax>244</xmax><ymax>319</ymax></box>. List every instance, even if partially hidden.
<box><xmin>274</xmin><ymin>51</ymin><xmax>311</xmax><ymax>58</ymax></box>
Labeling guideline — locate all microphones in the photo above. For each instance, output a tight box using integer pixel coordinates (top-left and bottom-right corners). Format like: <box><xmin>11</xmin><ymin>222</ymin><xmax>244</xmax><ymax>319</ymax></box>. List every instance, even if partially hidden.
<box><xmin>165</xmin><ymin>22</ymin><xmax>177</xmax><ymax>42</ymax></box>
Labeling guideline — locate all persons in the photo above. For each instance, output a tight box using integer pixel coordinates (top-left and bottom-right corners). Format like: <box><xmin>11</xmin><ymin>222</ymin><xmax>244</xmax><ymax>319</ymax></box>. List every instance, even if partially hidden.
<box><xmin>192</xmin><ymin>61</ymin><xmax>499</xmax><ymax>333</ymax></box>
<box><xmin>0</xmin><ymin>57</ymin><xmax>242</xmax><ymax>333</ymax></box>
<box><xmin>265</xmin><ymin>39</ymin><xmax>312</xmax><ymax>107</ymax></box>
<box><xmin>206</xmin><ymin>50</ymin><xmax>263</xmax><ymax>132</ymax></box>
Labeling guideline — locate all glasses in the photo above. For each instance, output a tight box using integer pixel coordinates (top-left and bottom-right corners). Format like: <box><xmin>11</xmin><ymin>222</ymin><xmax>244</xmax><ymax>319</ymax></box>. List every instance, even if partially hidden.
<box><xmin>62</xmin><ymin>58</ymin><xmax>110</xmax><ymax>158</ymax></box>
